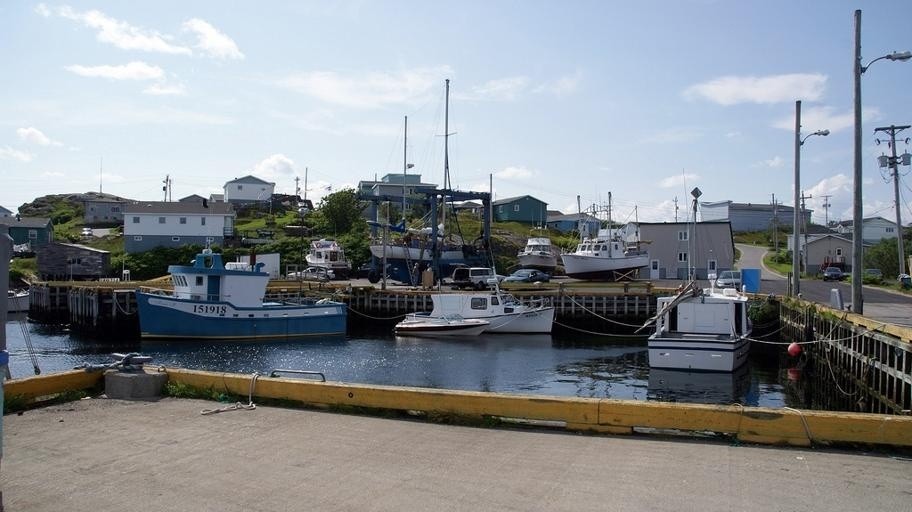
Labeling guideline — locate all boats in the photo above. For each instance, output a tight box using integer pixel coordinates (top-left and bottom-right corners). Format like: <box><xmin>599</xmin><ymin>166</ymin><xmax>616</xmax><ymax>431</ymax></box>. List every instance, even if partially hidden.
<box><xmin>136</xmin><ymin>237</ymin><xmax>349</xmax><ymax>342</ymax></box>
<box><xmin>392</xmin><ymin>293</ymin><xmax>557</xmax><ymax>337</ymax></box>
<box><xmin>518</xmin><ymin>192</ymin><xmax>652</xmax><ymax>279</ymax></box>
<box><xmin>634</xmin><ymin>188</ymin><xmax>755</xmax><ymax>372</ymax></box>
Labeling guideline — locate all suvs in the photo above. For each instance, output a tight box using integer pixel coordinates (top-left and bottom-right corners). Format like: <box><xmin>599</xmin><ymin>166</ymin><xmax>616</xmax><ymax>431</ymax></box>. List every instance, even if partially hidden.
<box><xmin>442</xmin><ymin>267</ymin><xmax>507</xmax><ymax>289</ymax></box>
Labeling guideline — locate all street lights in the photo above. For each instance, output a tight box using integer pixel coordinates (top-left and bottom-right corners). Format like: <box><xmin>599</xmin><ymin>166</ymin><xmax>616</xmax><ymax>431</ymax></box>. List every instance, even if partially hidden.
<box><xmin>851</xmin><ymin>10</ymin><xmax>911</xmax><ymax>316</ymax></box>
<box><xmin>792</xmin><ymin>99</ymin><xmax>830</xmax><ymax>297</ymax></box>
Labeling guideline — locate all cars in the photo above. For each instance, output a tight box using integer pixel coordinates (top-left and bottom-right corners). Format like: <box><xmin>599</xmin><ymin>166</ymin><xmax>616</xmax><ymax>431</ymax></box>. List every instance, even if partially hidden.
<box><xmin>80</xmin><ymin>228</ymin><xmax>93</xmax><ymax>236</ymax></box>
<box><xmin>502</xmin><ymin>268</ymin><xmax>550</xmax><ymax>283</ymax></box>
<box><xmin>822</xmin><ymin>267</ymin><xmax>844</xmax><ymax>281</ymax></box>
<box><xmin>864</xmin><ymin>269</ymin><xmax>884</xmax><ymax>281</ymax></box>
<box><xmin>716</xmin><ymin>270</ymin><xmax>742</xmax><ymax>291</ymax></box>
